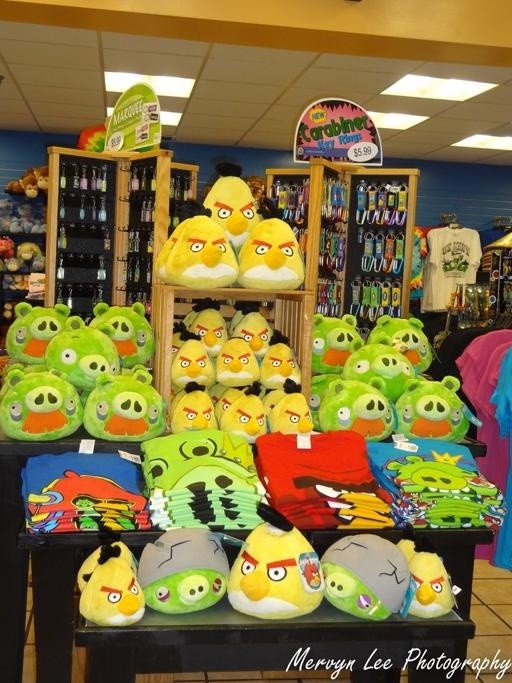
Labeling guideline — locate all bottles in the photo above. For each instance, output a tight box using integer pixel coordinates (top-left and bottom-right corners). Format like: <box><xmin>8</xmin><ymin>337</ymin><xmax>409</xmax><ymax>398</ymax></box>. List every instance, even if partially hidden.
<box><xmin>56</xmin><ymin>161</ymin><xmax>189</xmax><ymax>324</ymax></box>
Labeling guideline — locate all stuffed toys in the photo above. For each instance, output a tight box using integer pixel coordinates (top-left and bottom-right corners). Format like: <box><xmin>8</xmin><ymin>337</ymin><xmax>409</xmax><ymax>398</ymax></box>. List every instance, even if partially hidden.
<box><xmin>0</xmin><ymin>155</ymin><xmax>470</xmax><ymax>630</ymax></box>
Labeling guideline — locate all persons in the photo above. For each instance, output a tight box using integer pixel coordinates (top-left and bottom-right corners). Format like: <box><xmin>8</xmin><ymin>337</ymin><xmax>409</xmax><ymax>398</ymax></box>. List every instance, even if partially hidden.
<box><xmin>0</xmin><ymin>155</ymin><xmax>470</xmax><ymax>630</ymax></box>
